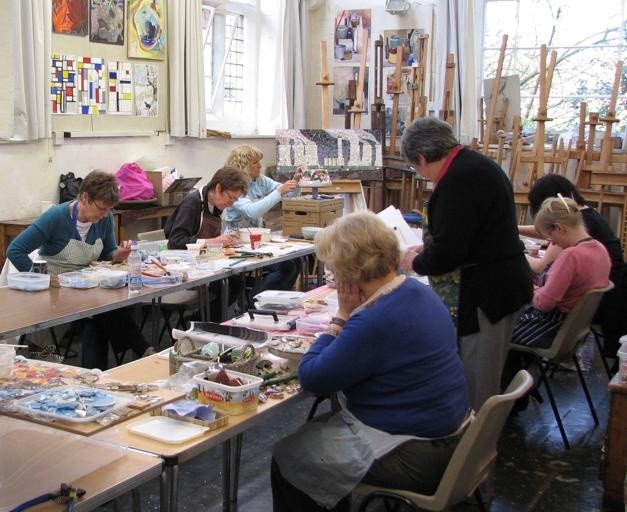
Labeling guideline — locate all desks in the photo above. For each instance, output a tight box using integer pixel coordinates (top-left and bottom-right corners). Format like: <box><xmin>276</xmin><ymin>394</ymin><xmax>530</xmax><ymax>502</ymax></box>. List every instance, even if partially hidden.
<box><xmin>0</xmin><ymin>413</ymin><xmax>170</xmax><ymax>511</ymax></box>
<box><xmin>1</xmin><ymin>204</ymin><xmax>184</xmax><ymax>260</ymax></box>
<box><xmin>0</xmin><ymin>227</ymin><xmax>549</xmax><ymax>512</ymax></box>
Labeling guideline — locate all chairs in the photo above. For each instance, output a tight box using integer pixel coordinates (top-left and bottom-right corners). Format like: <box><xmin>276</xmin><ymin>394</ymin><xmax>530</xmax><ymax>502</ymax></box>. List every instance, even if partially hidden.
<box><xmin>508</xmin><ymin>281</ymin><xmax>616</xmax><ymax>449</ymax></box>
<box><xmin>346</xmin><ymin>369</ymin><xmax>534</xmax><ymax>512</ymax></box>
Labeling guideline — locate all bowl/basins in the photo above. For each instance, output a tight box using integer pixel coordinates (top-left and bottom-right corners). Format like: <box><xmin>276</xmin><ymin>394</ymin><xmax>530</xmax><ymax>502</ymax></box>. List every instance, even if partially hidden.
<box><xmin>302</xmin><ymin>227</ymin><xmax>323</xmax><ymax>240</ymax></box>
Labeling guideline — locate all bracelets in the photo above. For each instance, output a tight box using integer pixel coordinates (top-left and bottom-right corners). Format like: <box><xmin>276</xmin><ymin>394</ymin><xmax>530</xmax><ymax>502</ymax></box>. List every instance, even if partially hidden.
<box><xmin>330</xmin><ymin>317</ymin><xmax>346</xmax><ymax>327</ymax></box>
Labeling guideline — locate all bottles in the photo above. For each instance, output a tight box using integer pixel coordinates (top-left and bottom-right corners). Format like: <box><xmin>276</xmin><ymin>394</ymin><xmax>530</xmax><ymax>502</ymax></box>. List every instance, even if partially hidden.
<box><xmin>127</xmin><ymin>245</ymin><xmax>143</xmax><ymax>298</ymax></box>
<box><xmin>33</xmin><ymin>260</ymin><xmax>47</xmax><ymax>273</ymax></box>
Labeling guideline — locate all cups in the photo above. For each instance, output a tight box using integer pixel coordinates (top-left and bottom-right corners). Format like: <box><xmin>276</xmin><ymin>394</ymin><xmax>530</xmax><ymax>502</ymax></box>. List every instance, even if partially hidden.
<box><xmin>186</xmin><ymin>233</ymin><xmax>263</xmax><ymax>261</ymax></box>
<box><xmin>0</xmin><ymin>346</ymin><xmax>15</xmax><ymax>378</ymax></box>
<box><xmin>528</xmin><ymin>246</ymin><xmax>539</xmax><ymax>257</ymax></box>
<box><xmin>42</xmin><ymin>201</ymin><xmax>56</xmax><ymax>213</ymax></box>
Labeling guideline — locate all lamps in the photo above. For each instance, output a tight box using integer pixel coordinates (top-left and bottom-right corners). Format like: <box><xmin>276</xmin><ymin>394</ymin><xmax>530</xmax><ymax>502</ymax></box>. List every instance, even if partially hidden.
<box><xmin>385</xmin><ymin>0</ymin><xmax>412</xmax><ymax>19</ymax></box>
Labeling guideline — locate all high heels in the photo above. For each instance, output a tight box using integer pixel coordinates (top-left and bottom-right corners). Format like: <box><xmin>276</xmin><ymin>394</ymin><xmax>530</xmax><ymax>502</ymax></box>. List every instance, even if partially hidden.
<box><xmin>510</xmin><ymin>386</ymin><xmax>543</xmax><ymax>411</ymax></box>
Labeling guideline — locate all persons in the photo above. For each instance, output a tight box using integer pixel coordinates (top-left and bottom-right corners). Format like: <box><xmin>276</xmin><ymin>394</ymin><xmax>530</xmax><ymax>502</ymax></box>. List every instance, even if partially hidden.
<box><xmin>5</xmin><ymin>168</ymin><xmax>156</xmax><ymax>371</ymax></box>
<box><xmin>224</xmin><ymin>144</ymin><xmax>308</xmax><ymax>317</ymax></box>
<box><xmin>399</xmin><ymin>116</ymin><xmax>534</xmax><ymax>512</ymax></box>
<box><xmin>517</xmin><ymin>172</ymin><xmax>627</xmax><ymax>374</ymax></box>
<box><xmin>500</xmin><ymin>192</ymin><xmax>613</xmax><ymax>417</ymax></box>
<box><xmin>164</xmin><ymin>165</ymin><xmax>249</xmax><ymax>331</ymax></box>
<box><xmin>271</xmin><ymin>210</ymin><xmax>476</xmax><ymax>512</ymax></box>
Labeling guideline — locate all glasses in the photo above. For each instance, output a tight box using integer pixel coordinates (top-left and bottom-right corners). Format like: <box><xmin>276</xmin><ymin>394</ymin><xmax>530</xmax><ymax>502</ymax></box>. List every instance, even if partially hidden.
<box><xmin>87</xmin><ymin>194</ymin><xmax>114</xmax><ymax>212</ymax></box>
<box><xmin>322</xmin><ymin>270</ymin><xmax>337</xmax><ymax>284</ymax></box>
<box><xmin>409</xmin><ymin>157</ymin><xmax>419</xmax><ymax>171</ymax></box>
<box><xmin>543</xmin><ymin>225</ymin><xmax>555</xmax><ymax>241</ymax></box>
<box><xmin>225</xmin><ymin>189</ymin><xmax>239</xmax><ymax>202</ymax></box>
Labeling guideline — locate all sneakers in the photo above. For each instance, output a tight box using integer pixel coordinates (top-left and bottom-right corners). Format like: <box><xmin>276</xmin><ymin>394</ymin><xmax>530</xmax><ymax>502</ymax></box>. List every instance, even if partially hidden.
<box><xmin>141</xmin><ymin>346</ymin><xmax>157</xmax><ymax>359</ymax></box>
